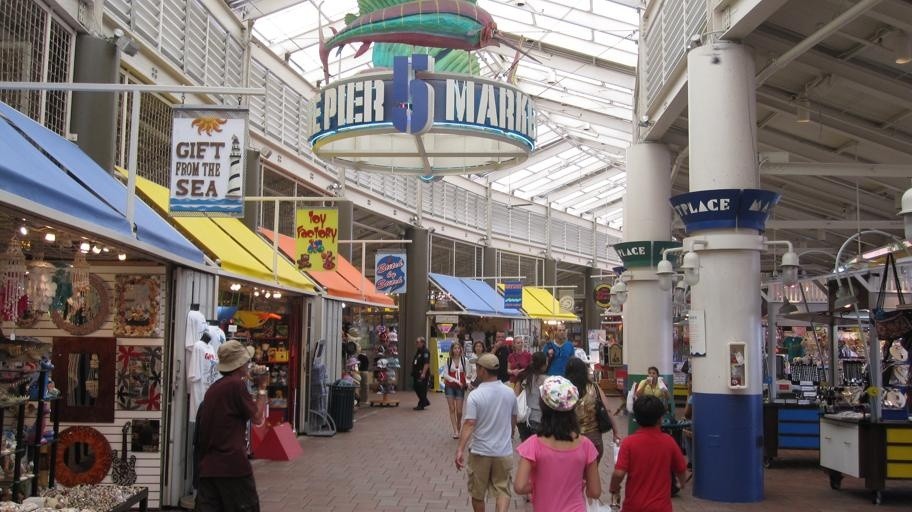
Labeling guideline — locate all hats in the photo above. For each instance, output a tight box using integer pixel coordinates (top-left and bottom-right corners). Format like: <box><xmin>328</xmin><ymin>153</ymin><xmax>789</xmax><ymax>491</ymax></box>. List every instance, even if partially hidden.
<box><xmin>386</xmin><ymin>384</ymin><xmax>397</xmax><ymax>395</ymax></box>
<box><xmin>345</xmin><ymin>357</ymin><xmax>360</xmax><ymax>368</ymax></box>
<box><xmin>377</xmin><ymin>372</ymin><xmax>388</xmax><ymax>382</ymax></box>
<box><xmin>388</xmin><ymin>358</ymin><xmax>400</xmax><ymax>368</ymax></box>
<box><xmin>386</xmin><ymin>370</ymin><xmax>397</xmax><ymax>381</ymax></box>
<box><xmin>376</xmin><ymin>345</ymin><xmax>384</xmax><ymax>356</ymax></box>
<box><xmin>469</xmin><ymin>353</ymin><xmax>500</xmax><ymax>370</ymax></box>
<box><xmin>346</xmin><ymin>328</ymin><xmax>362</xmax><ymax>341</ymax></box>
<box><xmin>352</xmin><ymin>341</ymin><xmax>361</xmax><ymax>356</ymax></box>
<box><xmin>538</xmin><ymin>375</ymin><xmax>579</xmax><ymax>412</ymax></box>
<box><xmin>214</xmin><ymin>339</ymin><xmax>255</xmax><ymax>372</ymax></box>
<box><xmin>388</xmin><ymin>345</ymin><xmax>398</xmax><ymax>357</ymax></box>
<box><xmin>375</xmin><ymin>326</ymin><xmax>387</xmax><ymax>341</ymax></box>
<box><xmin>377</xmin><ymin>384</ymin><xmax>386</xmax><ymax>395</ymax></box>
<box><xmin>386</xmin><ymin>332</ymin><xmax>397</xmax><ymax>343</ymax></box>
<box><xmin>376</xmin><ymin>359</ymin><xmax>388</xmax><ymax>369</ymax></box>
<box><xmin>342</xmin><ymin>375</ymin><xmax>354</xmax><ymax>384</ymax></box>
<box><xmin>345</xmin><ymin>341</ymin><xmax>356</xmax><ymax>355</ymax></box>
<box><xmin>352</xmin><ymin>373</ymin><xmax>361</xmax><ymax>385</ymax></box>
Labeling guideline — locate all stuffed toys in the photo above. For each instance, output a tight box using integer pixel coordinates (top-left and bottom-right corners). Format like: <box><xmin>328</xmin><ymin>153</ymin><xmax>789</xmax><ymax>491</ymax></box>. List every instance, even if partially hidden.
<box><xmin>0</xmin><ymin>329</ymin><xmax>65</xmax><ymax>503</ymax></box>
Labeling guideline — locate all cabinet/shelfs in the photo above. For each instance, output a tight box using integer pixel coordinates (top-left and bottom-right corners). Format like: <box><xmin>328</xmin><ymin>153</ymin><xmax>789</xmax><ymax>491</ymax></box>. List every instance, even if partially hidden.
<box><xmin>249</xmin><ymin>334</ymin><xmax>289</xmax><ymax>411</ymax></box>
<box><xmin>668</xmin><ymin>368</ymin><xmax>912</xmax><ymax>505</ymax></box>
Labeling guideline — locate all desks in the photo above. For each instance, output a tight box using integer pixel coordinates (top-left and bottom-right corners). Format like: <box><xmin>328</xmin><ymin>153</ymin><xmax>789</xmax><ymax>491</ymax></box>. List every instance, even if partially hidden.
<box><xmin>98</xmin><ymin>485</ymin><xmax>152</xmax><ymax>511</ymax></box>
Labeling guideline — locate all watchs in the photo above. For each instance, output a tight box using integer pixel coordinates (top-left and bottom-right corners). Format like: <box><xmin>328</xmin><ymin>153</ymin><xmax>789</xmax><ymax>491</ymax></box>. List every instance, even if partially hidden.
<box><xmin>256</xmin><ymin>388</ymin><xmax>266</xmax><ymax>396</ymax></box>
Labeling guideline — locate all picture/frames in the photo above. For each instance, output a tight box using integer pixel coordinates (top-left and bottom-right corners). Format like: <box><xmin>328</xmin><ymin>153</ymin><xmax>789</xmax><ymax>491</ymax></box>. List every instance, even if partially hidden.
<box><xmin>49</xmin><ymin>426</ymin><xmax>114</xmax><ymax>489</ymax></box>
<box><xmin>50</xmin><ymin>332</ymin><xmax>116</xmax><ymax>425</ymax></box>
<box><xmin>48</xmin><ymin>267</ymin><xmax>111</xmax><ymax>337</ymax></box>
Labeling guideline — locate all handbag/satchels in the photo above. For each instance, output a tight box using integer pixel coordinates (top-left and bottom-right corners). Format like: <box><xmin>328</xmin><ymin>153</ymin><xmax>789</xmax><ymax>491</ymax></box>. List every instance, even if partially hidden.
<box><xmin>626</xmin><ymin>382</ymin><xmax>637</xmax><ymax>414</ymax></box>
<box><xmin>515</xmin><ymin>361</ymin><xmax>521</xmax><ymax>370</ymax></box>
<box><xmin>876</xmin><ymin>309</ymin><xmax>912</xmax><ymax>340</ymax></box>
<box><xmin>516</xmin><ymin>380</ymin><xmax>531</xmax><ymax>424</ymax></box>
<box><xmin>681</xmin><ymin>361</ymin><xmax>689</xmax><ymax>374</ymax></box>
<box><xmin>596</xmin><ymin>400</ymin><xmax>612</xmax><ymax>433</ymax></box>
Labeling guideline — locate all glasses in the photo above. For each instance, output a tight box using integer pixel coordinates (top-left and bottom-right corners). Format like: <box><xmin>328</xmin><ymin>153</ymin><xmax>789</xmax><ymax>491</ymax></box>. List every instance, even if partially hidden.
<box><xmin>416</xmin><ymin>341</ymin><xmax>422</xmax><ymax>344</ymax></box>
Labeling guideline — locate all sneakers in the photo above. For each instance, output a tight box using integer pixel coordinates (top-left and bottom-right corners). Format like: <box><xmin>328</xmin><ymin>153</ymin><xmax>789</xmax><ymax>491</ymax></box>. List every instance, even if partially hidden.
<box><xmin>687</xmin><ymin>462</ymin><xmax>693</xmax><ymax>472</ymax></box>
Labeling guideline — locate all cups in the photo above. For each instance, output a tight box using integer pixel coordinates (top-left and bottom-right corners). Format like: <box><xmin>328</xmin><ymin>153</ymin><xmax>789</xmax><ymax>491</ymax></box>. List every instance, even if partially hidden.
<box><xmin>647</xmin><ymin>376</ymin><xmax>653</xmax><ymax>385</ymax></box>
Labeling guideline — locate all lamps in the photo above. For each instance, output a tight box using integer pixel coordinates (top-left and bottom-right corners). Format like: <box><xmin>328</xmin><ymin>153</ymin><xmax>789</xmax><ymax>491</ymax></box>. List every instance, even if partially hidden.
<box><xmin>671</xmin><ymin>273</ymin><xmax>689</xmax><ymax>303</ymax></box>
<box><xmin>764</xmin><ymin>239</ymin><xmax>802</xmax><ymax>285</ymax></box>
<box><xmin>679</xmin><ymin>238</ymin><xmax>708</xmax><ymax>286</ymax></box>
<box><xmin>19</xmin><ymin>215</ymin><xmax>128</xmax><ymax>264</ymax></box>
<box><xmin>614</xmin><ymin>273</ymin><xmax>633</xmax><ymax>303</ymax></box>
<box><xmin>608</xmin><ymin>278</ymin><xmax>620</xmax><ymax>308</ymax></box>
<box><xmin>655</xmin><ymin>246</ymin><xmax>684</xmax><ymax>291</ymax></box>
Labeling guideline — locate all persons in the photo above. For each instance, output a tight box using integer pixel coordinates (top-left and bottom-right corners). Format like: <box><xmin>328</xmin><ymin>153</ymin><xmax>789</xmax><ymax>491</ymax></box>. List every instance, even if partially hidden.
<box><xmin>683</xmin><ymin>374</ymin><xmax>692</xmax><ymax>419</ymax></box>
<box><xmin>442</xmin><ymin>341</ymin><xmax>471</xmax><ymax>439</ymax></box>
<box><xmin>513</xmin><ymin>351</ymin><xmax>551</xmax><ymax>442</ymax></box>
<box><xmin>635</xmin><ymin>366</ymin><xmax>672</xmax><ymax>401</ymax></box>
<box><xmin>609</xmin><ymin>394</ymin><xmax>688</xmax><ymax>510</ymax></box>
<box><xmin>563</xmin><ymin>357</ymin><xmax>623</xmax><ymax>465</ymax></box>
<box><xmin>513</xmin><ymin>374</ymin><xmax>601</xmax><ymax>512</ymax></box>
<box><xmin>542</xmin><ymin>322</ymin><xmax>575</xmax><ymax>374</ymax></box>
<box><xmin>487</xmin><ymin>330</ymin><xmax>514</xmax><ymax>381</ymax></box>
<box><xmin>507</xmin><ymin>336</ymin><xmax>533</xmax><ymax>380</ymax></box>
<box><xmin>412</xmin><ymin>335</ymin><xmax>431</xmax><ymax>409</ymax></box>
<box><xmin>455</xmin><ymin>351</ymin><xmax>517</xmax><ymax>511</ymax></box>
<box><xmin>192</xmin><ymin>340</ymin><xmax>269</xmax><ymax>512</ymax></box>
<box><xmin>468</xmin><ymin>340</ymin><xmax>487</xmax><ymax>392</ymax></box>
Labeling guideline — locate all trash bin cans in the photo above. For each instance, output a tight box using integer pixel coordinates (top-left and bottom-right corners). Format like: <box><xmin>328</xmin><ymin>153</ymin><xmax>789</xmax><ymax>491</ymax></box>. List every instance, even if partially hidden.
<box><xmin>662</xmin><ymin>422</ymin><xmax>692</xmax><ymax>495</ymax></box>
<box><xmin>325</xmin><ymin>379</ymin><xmax>361</xmax><ymax>432</ymax></box>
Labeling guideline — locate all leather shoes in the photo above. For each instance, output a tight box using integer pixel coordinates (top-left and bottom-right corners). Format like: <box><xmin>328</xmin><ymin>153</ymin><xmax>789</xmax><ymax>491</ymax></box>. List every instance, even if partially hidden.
<box><xmin>457</xmin><ymin>429</ymin><xmax>461</xmax><ymax>438</ymax></box>
<box><xmin>453</xmin><ymin>430</ymin><xmax>458</xmax><ymax>439</ymax></box>
<box><xmin>413</xmin><ymin>406</ymin><xmax>424</xmax><ymax>410</ymax></box>
<box><xmin>418</xmin><ymin>402</ymin><xmax>430</xmax><ymax>407</ymax></box>
